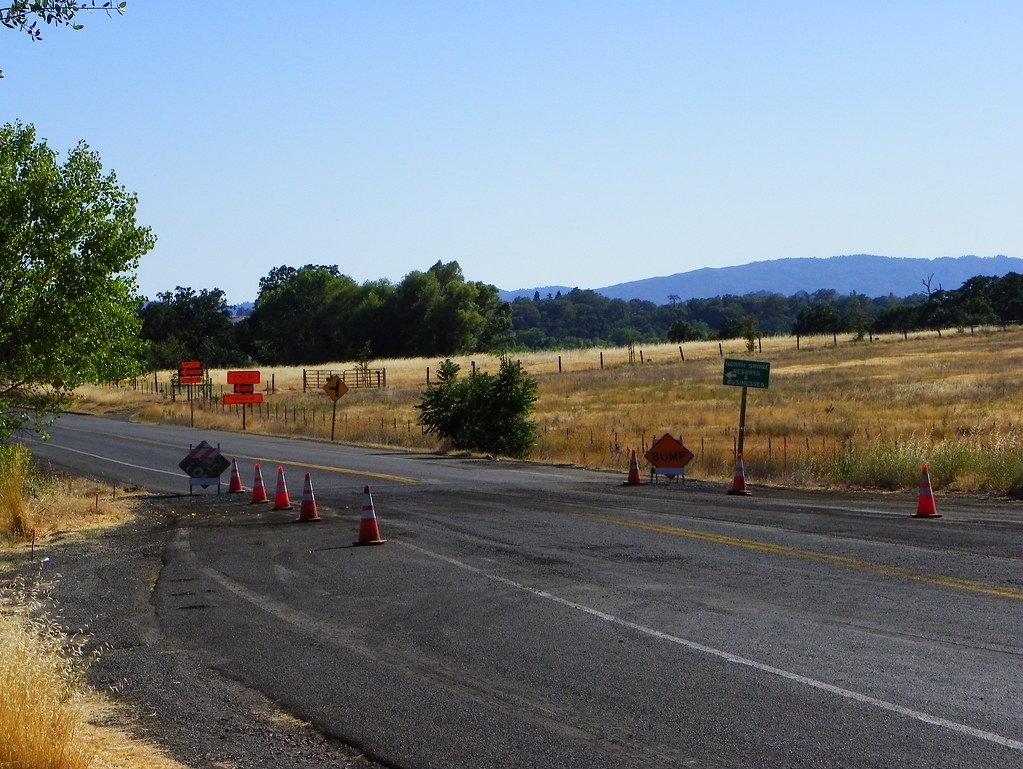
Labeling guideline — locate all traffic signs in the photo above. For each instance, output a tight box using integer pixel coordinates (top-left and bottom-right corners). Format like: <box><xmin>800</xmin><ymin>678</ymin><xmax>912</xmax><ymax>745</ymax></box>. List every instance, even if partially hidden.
<box><xmin>234</xmin><ymin>384</ymin><xmax>253</xmax><ymax>393</ymax></box>
<box><xmin>178</xmin><ymin>368</ymin><xmax>204</xmax><ymax>377</ymax></box>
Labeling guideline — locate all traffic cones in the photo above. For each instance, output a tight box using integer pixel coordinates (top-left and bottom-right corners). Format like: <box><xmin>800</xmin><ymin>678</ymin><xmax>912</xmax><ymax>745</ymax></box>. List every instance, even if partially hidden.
<box><xmin>272</xmin><ymin>467</ymin><xmax>293</xmax><ymax>510</ymax></box>
<box><xmin>910</xmin><ymin>462</ymin><xmax>943</xmax><ymax>518</ymax></box>
<box><xmin>248</xmin><ymin>464</ymin><xmax>270</xmax><ymax>504</ymax></box>
<box><xmin>352</xmin><ymin>486</ymin><xmax>387</xmax><ymax>545</ymax></box>
<box><xmin>296</xmin><ymin>472</ymin><xmax>323</xmax><ymax>521</ymax></box>
<box><xmin>623</xmin><ymin>450</ymin><xmax>645</xmax><ymax>486</ymax></box>
<box><xmin>227</xmin><ymin>457</ymin><xmax>245</xmax><ymax>493</ymax></box>
<box><xmin>727</xmin><ymin>453</ymin><xmax>753</xmax><ymax>496</ymax></box>
